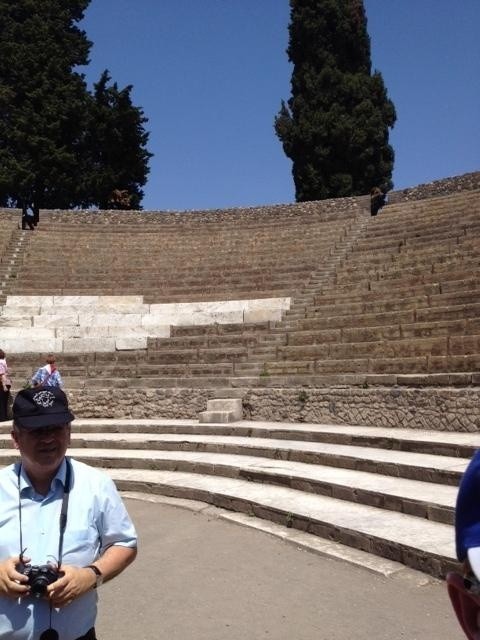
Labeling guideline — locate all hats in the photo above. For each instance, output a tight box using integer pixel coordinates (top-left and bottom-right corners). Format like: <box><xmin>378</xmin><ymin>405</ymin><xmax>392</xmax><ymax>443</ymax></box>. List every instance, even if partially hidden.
<box><xmin>12</xmin><ymin>387</ymin><xmax>74</xmax><ymax>429</ymax></box>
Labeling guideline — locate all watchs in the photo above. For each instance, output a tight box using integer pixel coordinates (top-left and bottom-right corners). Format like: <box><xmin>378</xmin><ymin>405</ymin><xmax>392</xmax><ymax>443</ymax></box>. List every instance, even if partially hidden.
<box><xmin>85</xmin><ymin>565</ymin><xmax>104</xmax><ymax>589</ymax></box>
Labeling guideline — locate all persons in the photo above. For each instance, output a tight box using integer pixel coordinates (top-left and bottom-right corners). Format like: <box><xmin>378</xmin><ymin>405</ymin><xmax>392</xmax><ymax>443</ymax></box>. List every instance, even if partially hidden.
<box><xmin>0</xmin><ymin>385</ymin><xmax>139</xmax><ymax>639</ymax></box>
<box><xmin>32</xmin><ymin>352</ymin><xmax>63</xmax><ymax>391</ymax></box>
<box><xmin>447</xmin><ymin>449</ymin><xmax>480</xmax><ymax>640</ymax></box>
<box><xmin>0</xmin><ymin>347</ymin><xmax>13</xmax><ymax>420</ymax></box>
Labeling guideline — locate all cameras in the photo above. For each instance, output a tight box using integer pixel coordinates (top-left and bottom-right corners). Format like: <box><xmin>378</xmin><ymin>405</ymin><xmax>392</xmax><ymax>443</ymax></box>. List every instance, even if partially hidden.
<box><xmin>20</xmin><ymin>564</ymin><xmax>63</xmax><ymax>599</ymax></box>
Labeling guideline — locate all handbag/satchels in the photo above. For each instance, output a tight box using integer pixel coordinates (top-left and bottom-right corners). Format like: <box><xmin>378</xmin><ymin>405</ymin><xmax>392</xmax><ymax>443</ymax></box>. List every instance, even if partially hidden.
<box><xmin>446</xmin><ymin>572</ymin><xmax>480</xmax><ymax>639</ymax></box>
